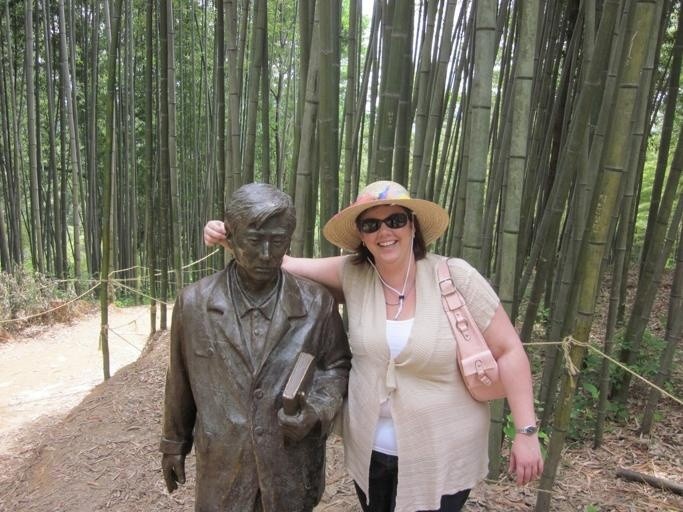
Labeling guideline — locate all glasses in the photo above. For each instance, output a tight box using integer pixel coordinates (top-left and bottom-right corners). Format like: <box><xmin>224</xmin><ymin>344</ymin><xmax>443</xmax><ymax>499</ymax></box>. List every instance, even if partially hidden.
<box><xmin>357</xmin><ymin>212</ymin><xmax>412</xmax><ymax>234</ymax></box>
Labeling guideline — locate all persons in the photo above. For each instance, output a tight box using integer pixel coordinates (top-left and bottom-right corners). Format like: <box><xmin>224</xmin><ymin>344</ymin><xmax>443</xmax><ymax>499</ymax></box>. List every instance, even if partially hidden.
<box><xmin>156</xmin><ymin>181</ymin><xmax>352</xmax><ymax>512</ymax></box>
<box><xmin>201</xmin><ymin>180</ymin><xmax>545</xmax><ymax>510</ymax></box>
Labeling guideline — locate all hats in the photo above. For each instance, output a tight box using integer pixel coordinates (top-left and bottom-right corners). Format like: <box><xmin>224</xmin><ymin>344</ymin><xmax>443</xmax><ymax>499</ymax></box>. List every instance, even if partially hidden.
<box><xmin>322</xmin><ymin>180</ymin><xmax>451</xmax><ymax>252</ymax></box>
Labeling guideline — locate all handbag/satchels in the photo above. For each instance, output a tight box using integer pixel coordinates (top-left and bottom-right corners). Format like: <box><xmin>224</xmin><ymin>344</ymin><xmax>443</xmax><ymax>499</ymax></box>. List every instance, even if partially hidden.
<box><xmin>437</xmin><ymin>254</ymin><xmax>510</xmax><ymax>403</ymax></box>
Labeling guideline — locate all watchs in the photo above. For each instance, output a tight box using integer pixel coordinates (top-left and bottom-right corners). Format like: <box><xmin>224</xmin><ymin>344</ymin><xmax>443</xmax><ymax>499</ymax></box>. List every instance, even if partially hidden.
<box><xmin>511</xmin><ymin>424</ymin><xmax>538</xmax><ymax>436</ymax></box>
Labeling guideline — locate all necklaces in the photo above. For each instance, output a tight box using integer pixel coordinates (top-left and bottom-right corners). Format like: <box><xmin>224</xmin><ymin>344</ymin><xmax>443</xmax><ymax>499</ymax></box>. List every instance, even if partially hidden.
<box><xmin>383</xmin><ymin>274</ymin><xmax>418</xmax><ymax>307</ymax></box>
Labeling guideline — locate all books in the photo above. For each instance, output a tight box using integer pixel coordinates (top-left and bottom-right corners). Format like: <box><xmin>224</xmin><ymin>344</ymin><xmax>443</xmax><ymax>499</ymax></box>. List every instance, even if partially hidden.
<box><xmin>278</xmin><ymin>350</ymin><xmax>317</xmax><ymax>451</ymax></box>
